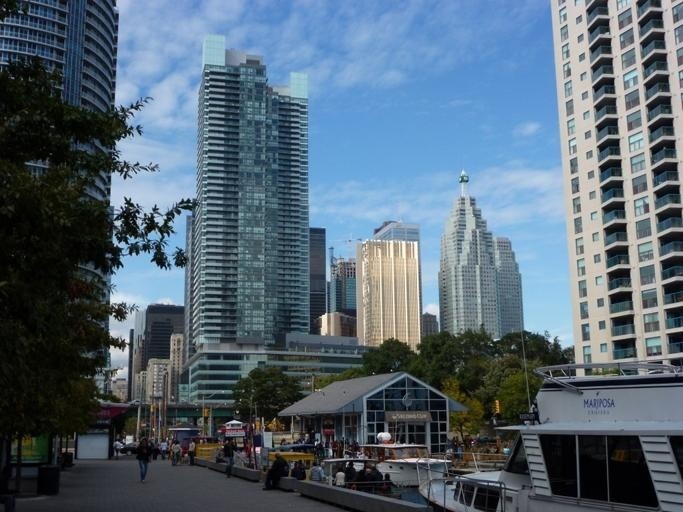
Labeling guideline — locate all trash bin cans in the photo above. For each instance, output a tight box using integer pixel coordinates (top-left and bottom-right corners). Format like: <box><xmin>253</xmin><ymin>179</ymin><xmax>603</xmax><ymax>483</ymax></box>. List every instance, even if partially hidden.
<box><xmin>37</xmin><ymin>464</ymin><xmax>60</xmax><ymax>495</ymax></box>
<box><xmin>63</xmin><ymin>453</ymin><xmax>73</xmax><ymax>466</ymax></box>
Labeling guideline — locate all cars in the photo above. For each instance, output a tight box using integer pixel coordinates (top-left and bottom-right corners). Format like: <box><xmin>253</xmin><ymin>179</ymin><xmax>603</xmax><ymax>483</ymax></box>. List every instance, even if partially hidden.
<box><xmin>120</xmin><ymin>442</ymin><xmax>160</xmax><ymax>456</ymax></box>
<box><xmin>129</xmin><ymin>400</ymin><xmax>144</xmax><ymax>406</ymax></box>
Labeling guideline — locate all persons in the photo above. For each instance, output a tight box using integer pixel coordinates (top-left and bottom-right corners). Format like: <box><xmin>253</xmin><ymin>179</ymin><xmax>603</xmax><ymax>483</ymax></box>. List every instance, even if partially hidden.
<box><xmin>135</xmin><ymin>436</ymin><xmax>195</xmax><ymax>482</ymax></box>
<box><xmin>112</xmin><ymin>438</ymin><xmax>121</xmax><ymax>457</ymax></box>
<box><xmin>262</xmin><ymin>436</ymin><xmax>396</xmax><ymax>494</ymax></box>
<box><xmin>446</xmin><ymin>434</ymin><xmax>477</xmax><ymax>462</ymax></box>
<box><xmin>222</xmin><ymin>435</ymin><xmax>237</xmax><ymax>479</ymax></box>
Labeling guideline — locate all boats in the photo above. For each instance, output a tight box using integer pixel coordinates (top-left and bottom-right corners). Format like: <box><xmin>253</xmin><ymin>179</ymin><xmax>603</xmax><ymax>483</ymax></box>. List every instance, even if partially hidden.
<box><xmin>271</xmin><ymin>444</ymin><xmax>452</xmax><ymax>500</ymax></box>
<box><xmin>418</xmin><ymin>321</ymin><xmax>683</xmax><ymax>512</ymax></box>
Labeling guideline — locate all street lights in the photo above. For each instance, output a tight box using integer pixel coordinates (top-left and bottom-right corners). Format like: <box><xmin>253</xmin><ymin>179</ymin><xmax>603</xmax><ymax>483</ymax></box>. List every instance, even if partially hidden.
<box><xmin>202</xmin><ymin>390</ymin><xmax>227</xmax><ymax>437</ymax></box>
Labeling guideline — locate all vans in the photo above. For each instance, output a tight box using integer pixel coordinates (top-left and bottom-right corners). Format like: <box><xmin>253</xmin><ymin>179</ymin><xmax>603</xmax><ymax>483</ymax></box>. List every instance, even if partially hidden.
<box><xmin>181</xmin><ymin>436</ymin><xmax>219</xmax><ymax>457</ymax></box>
